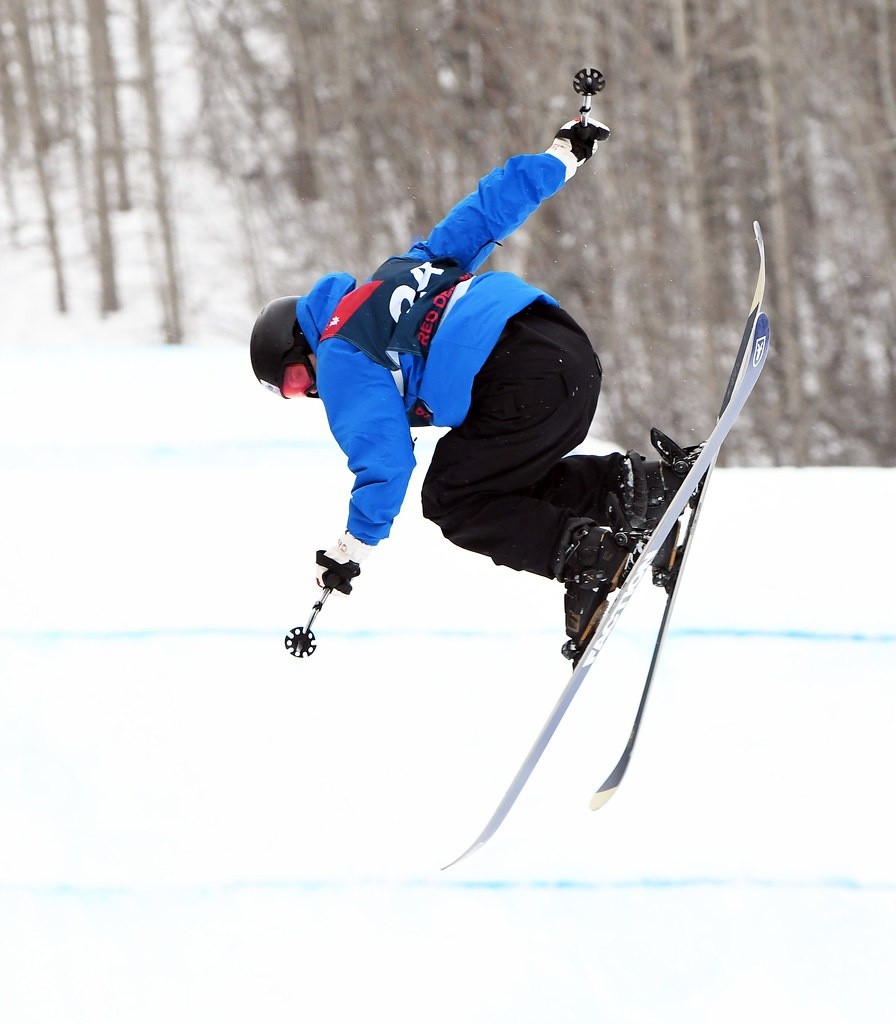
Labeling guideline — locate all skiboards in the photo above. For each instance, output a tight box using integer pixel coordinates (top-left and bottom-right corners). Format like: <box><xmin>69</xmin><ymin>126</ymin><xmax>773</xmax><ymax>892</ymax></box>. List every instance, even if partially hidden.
<box><xmin>433</xmin><ymin>211</ymin><xmax>777</xmax><ymax>878</ymax></box>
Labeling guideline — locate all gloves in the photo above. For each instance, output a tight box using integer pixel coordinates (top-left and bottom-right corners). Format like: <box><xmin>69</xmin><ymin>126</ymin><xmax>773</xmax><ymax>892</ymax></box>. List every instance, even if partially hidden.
<box><xmin>315</xmin><ymin>529</ymin><xmax>373</xmax><ymax>595</ymax></box>
<box><xmin>543</xmin><ymin>118</ymin><xmax>610</xmax><ymax>180</ymax></box>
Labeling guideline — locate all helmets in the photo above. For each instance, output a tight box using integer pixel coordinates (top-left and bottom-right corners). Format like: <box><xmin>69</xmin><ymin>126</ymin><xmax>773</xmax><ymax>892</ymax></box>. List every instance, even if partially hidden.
<box><xmin>250</xmin><ymin>293</ymin><xmax>304</xmax><ymax>400</ymax></box>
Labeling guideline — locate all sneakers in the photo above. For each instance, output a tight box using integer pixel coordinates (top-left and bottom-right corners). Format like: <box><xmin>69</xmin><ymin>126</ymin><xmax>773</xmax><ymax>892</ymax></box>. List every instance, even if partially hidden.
<box><xmin>563</xmin><ymin>524</ymin><xmax>638</xmax><ymax>657</ymax></box>
<box><xmin>626</xmin><ymin>428</ymin><xmax>705</xmax><ymax>587</ymax></box>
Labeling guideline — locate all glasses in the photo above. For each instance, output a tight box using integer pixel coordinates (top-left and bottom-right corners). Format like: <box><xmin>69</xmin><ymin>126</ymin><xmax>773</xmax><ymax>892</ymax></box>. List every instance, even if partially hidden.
<box><xmin>280</xmin><ymin>350</ymin><xmax>316</xmax><ymax>402</ymax></box>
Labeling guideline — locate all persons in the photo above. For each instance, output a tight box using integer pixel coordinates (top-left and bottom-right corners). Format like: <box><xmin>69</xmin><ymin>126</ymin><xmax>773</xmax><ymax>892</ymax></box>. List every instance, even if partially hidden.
<box><xmin>250</xmin><ymin>116</ymin><xmax>682</xmax><ymax>660</ymax></box>
